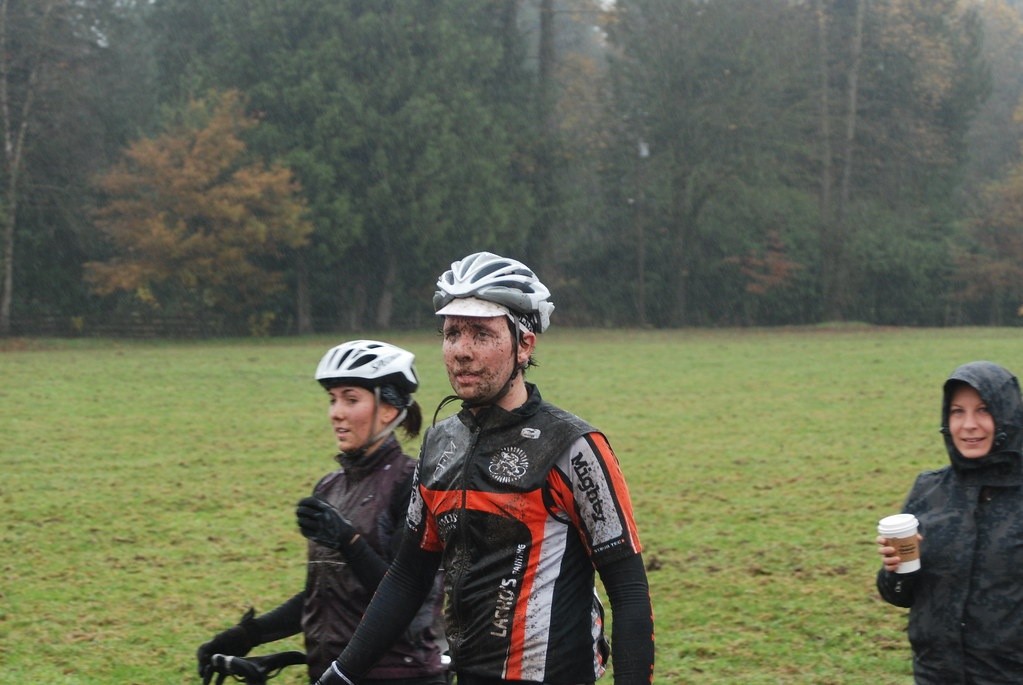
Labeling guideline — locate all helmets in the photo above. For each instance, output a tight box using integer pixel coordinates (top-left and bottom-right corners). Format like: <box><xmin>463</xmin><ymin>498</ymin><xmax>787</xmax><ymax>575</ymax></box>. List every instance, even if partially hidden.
<box><xmin>432</xmin><ymin>250</ymin><xmax>555</xmax><ymax>334</ymax></box>
<box><xmin>314</xmin><ymin>338</ymin><xmax>420</xmax><ymax>393</ymax></box>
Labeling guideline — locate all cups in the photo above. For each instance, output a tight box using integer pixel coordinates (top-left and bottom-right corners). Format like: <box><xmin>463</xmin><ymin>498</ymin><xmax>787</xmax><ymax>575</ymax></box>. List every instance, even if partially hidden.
<box><xmin>877</xmin><ymin>513</ymin><xmax>920</xmax><ymax>574</ymax></box>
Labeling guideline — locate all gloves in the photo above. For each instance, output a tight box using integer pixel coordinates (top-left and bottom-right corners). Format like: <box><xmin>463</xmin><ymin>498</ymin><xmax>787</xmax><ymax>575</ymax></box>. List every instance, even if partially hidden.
<box><xmin>295</xmin><ymin>495</ymin><xmax>355</xmax><ymax>549</ymax></box>
<box><xmin>198</xmin><ymin>607</ymin><xmax>261</xmax><ymax>677</ymax></box>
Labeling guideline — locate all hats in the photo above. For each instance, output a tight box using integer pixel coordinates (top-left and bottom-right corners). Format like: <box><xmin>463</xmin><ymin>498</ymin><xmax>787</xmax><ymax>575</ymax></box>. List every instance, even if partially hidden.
<box><xmin>436</xmin><ymin>298</ymin><xmax>538</xmax><ymax>334</ymax></box>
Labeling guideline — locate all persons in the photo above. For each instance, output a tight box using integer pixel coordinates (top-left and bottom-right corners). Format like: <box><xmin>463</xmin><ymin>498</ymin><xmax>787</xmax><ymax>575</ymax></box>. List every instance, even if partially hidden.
<box><xmin>197</xmin><ymin>340</ymin><xmax>455</xmax><ymax>685</ymax></box>
<box><xmin>316</xmin><ymin>252</ymin><xmax>656</xmax><ymax>685</ymax></box>
<box><xmin>877</xmin><ymin>361</ymin><xmax>1022</xmax><ymax>685</ymax></box>
